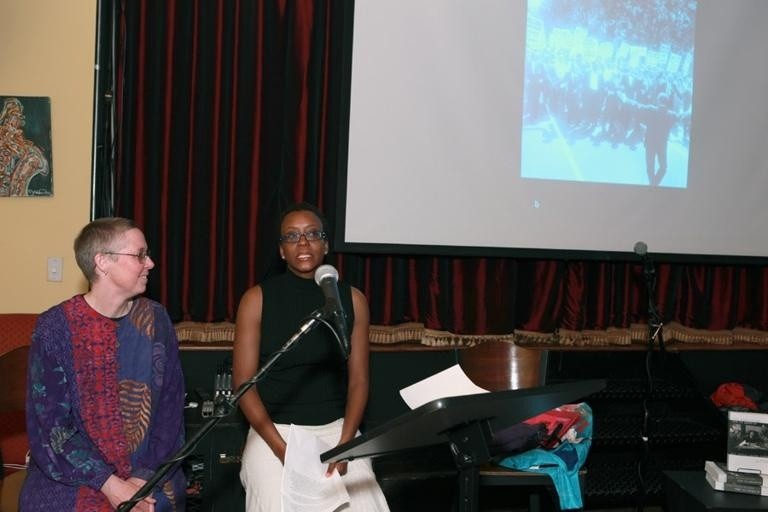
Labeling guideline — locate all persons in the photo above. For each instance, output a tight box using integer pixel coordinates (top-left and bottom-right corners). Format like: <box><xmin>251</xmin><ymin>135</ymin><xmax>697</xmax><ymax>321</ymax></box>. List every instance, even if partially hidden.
<box><xmin>737</xmin><ymin>427</ymin><xmax>765</xmax><ymax>453</ymax></box>
<box><xmin>231</xmin><ymin>208</ymin><xmax>392</xmax><ymax>512</ymax></box>
<box><xmin>17</xmin><ymin>216</ymin><xmax>189</xmax><ymax>511</ymax></box>
<box><xmin>522</xmin><ymin>0</ymin><xmax>697</xmax><ymax>190</ymax></box>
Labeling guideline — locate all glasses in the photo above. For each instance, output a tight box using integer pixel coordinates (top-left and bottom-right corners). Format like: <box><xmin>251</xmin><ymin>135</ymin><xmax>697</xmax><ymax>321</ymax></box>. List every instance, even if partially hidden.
<box><xmin>280</xmin><ymin>231</ymin><xmax>326</xmax><ymax>243</ymax></box>
<box><xmin>102</xmin><ymin>247</ymin><xmax>151</xmax><ymax>264</ymax></box>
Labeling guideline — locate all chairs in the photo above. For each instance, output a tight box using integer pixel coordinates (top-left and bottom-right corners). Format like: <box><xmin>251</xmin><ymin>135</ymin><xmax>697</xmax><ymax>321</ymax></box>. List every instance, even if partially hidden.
<box><xmin>455</xmin><ymin>341</ymin><xmax>587</xmax><ymax>512</ymax></box>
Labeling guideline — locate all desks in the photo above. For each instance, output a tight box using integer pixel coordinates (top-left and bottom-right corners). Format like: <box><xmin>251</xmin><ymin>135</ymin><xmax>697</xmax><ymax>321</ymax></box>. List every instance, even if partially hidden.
<box><xmin>654</xmin><ymin>470</ymin><xmax>768</xmax><ymax>512</ymax></box>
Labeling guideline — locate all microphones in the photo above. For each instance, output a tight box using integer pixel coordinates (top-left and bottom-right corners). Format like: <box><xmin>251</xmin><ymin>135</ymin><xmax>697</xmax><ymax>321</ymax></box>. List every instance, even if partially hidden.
<box><xmin>313</xmin><ymin>263</ymin><xmax>358</xmax><ymax>354</ymax></box>
<box><xmin>631</xmin><ymin>241</ymin><xmax>662</xmax><ymax>292</ymax></box>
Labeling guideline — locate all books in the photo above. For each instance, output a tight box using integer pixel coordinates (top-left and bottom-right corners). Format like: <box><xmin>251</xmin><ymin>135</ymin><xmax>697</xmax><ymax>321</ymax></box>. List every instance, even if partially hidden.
<box><xmin>726</xmin><ymin>409</ymin><xmax>768</xmax><ymax>476</ymax></box>
<box><xmin>705</xmin><ymin>459</ymin><xmax>768</xmax><ymax>496</ymax></box>
